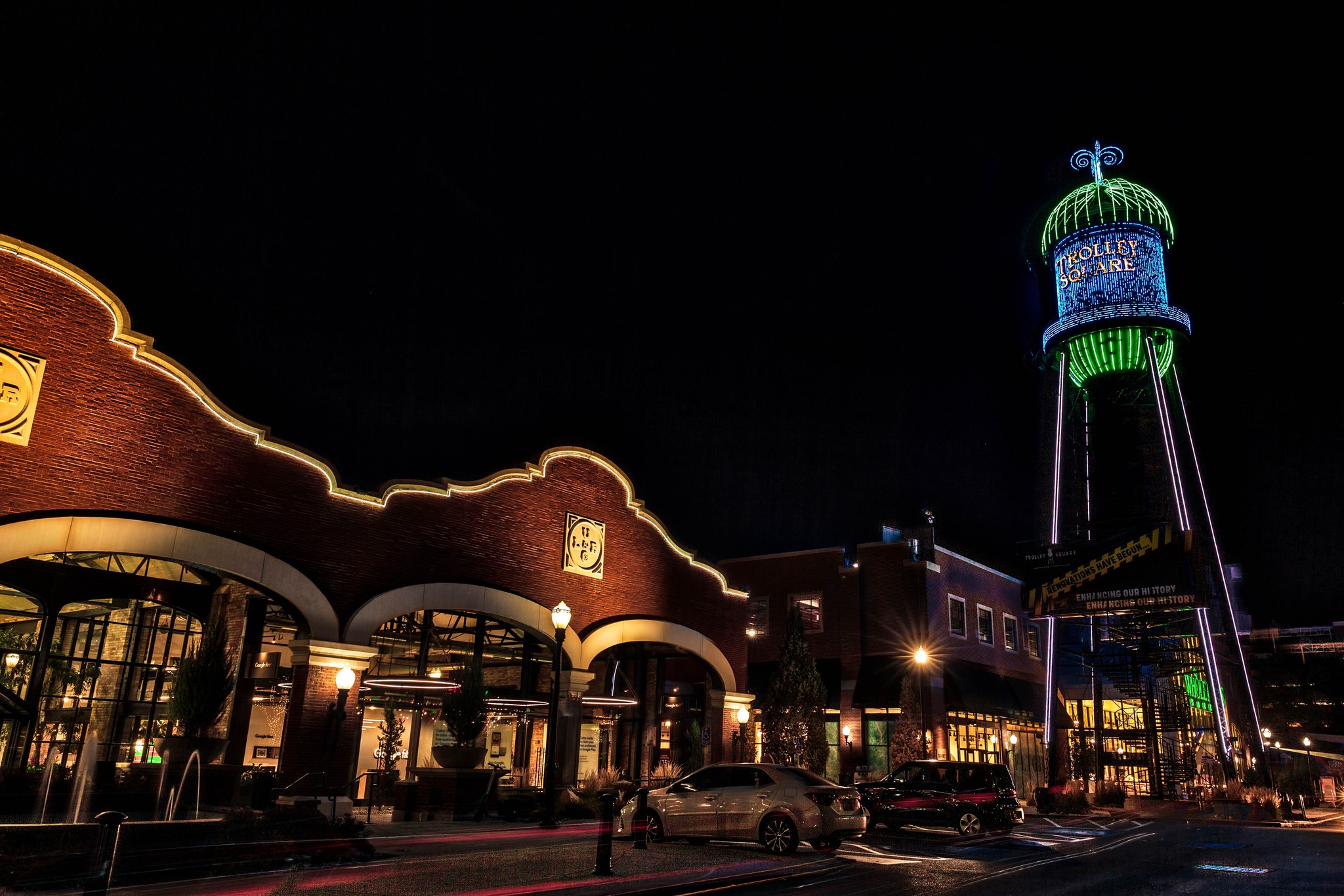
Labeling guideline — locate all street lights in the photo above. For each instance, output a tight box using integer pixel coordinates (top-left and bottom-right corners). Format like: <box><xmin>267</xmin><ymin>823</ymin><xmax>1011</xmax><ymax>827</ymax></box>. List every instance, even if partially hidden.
<box><xmin>737</xmin><ymin>704</ymin><xmax>749</xmax><ymax>762</ymax></box>
<box><xmin>326</xmin><ymin>667</ymin><xmax>358</xmax><ymax>796</ymax></box>
<box><xmin>1274</xmin><ymin>741</ymin><xmax>1287</xmax><ymax>802</ymax></box>
<box><xmin>1262</xmin><ymin>728</ymin><xmax>1276</xmax><ymax>792</ymax></box>
<box><xmin>537</xmin><ymin>599</ymin><xmax>575</xmax><ymax>828</ymax></box>
<box><xmin>913</xmin><ymin>644</ymin><xmax>931</xmax><ymax>759</ymax></box>
<box><xmin>1303</xmin><ymin>737</ymin><xmax>1316</xmax><ymax>797</ymax></box>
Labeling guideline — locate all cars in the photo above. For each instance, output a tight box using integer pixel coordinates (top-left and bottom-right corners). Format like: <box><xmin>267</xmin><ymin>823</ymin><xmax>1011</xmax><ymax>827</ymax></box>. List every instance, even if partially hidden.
<box><xmin>616</xmin><ymin>763</ymin><xmax>867</xmax><ymax>856</ymax></box>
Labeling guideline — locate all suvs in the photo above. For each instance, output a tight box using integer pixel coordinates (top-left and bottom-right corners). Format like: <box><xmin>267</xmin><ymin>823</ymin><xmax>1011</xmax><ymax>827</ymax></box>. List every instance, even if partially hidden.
<box><xmin>844</xmin><ymin>758</ymin><xmax>1024</xmax><ymax>841</ymax></box>
